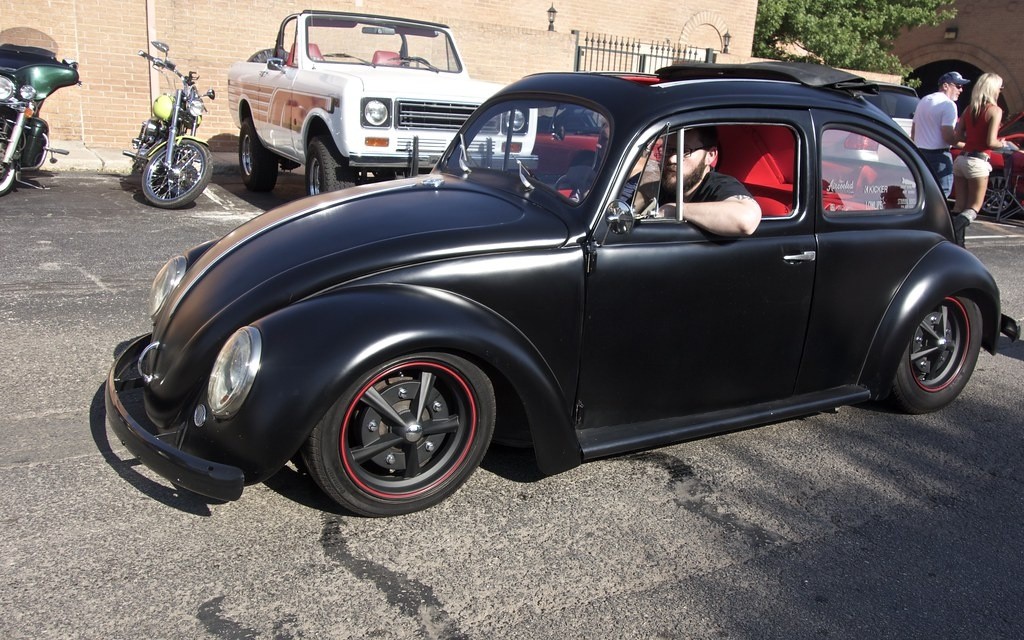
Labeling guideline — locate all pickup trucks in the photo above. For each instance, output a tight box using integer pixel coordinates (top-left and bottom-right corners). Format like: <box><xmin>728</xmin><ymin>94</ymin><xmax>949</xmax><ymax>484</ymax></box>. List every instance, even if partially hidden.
<box><xmin>227</xmin><ymin>9</ymin><xmax>540</xmax><ymax>199</ymax></box>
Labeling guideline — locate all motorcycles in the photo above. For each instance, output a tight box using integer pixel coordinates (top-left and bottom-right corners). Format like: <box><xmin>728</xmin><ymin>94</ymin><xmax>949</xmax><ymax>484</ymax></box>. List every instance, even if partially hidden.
<box><xmin>0</xmin><ymin>42</ymin><xmax>81</xmax><ymax>196</ymax></box>
<box><xmin>119</xmin><ymin>39</ymin><xmax>216</xmax><ymax>210</ymax></box>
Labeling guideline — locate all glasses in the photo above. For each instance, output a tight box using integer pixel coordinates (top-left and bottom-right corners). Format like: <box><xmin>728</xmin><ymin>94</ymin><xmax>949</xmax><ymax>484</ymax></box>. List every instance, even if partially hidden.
<box><xmin>1000</xmin><ymin>85</ymin><xmax>1004</xmax><ymax>91</ymax></box>
<box><xmin>952</xmin><ymin>82</ymin><xmax>963</xmax><ymax>89</ymax></box>
<box><xmin>658</xmin><ymin>145</ymin><xmax>706</xmax><ymax>159</ymax></box>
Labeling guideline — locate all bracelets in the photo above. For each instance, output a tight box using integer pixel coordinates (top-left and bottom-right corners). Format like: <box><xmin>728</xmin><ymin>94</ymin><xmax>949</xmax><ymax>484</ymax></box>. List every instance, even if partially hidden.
<box><xmin>999</xmin><ymin>140</ymin><xmax>1005</xmax><ymax>148</ymax></box>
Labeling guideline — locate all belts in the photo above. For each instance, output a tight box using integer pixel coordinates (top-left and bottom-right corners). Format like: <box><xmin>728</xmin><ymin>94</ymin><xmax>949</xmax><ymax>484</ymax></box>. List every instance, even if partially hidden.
<box><xmin>959</xmin><ymin>151</ymin><xmax>990</xmax><ymax>163</ymax></box>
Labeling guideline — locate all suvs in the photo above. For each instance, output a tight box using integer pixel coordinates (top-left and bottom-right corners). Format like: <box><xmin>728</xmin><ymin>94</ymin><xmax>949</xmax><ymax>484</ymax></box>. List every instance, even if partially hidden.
<box><xmin>819</xmin><ymin>81</ymin><xmax>925</xmax><ymax>193</ymax></box>
<box><xmin>533</xmin><ymin>71</ymin><xmax>668</xmax><ymax>186</ymax></box>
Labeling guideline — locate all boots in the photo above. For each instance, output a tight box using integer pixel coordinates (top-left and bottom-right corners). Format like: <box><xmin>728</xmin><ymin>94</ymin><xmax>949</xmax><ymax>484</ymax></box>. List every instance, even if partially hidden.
<box><xmin>952</xmin><ymin>208</ymin><xmax>978</xmax><ymax>233</ymax></box>
<box><xmin>950</xmin><ymin>210</ymin><xmax>966</xmax><ymax>249</ymax></box>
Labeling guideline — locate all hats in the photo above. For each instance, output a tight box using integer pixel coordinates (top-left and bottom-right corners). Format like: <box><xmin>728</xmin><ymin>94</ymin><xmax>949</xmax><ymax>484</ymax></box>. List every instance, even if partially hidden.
<box><xmin>938</xmin><ymin>71</ymin><xmax>970</xmax><ymax>86</ymax></box>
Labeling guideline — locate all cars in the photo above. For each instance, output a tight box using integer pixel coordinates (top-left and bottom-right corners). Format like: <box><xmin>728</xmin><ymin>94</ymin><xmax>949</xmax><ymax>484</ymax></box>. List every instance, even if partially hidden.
<box><xmin>103</xmin><ymin>58</ymin><xmax>1018</xmax><ymax>522</ymax></box>
<box><xmin>948</xmin><ymin>134</ymin><xmax>1024</xmax><ymax>218</ymax></box>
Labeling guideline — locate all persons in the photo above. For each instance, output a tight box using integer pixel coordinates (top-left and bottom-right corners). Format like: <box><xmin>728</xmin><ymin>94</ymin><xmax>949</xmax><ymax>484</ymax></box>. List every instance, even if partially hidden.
<box><xmin>911</xmin><ymin>71</ymin><xmax>967</xmax><ymax>199</ymax></box>
<box><xmin>951</xmin><ymin>72</ymin><xmax>1011</xmax><ymax>249</ymax></box>
<box><xmin>583</xmin><ymin>120</ymin><xmax>762</xmax><ymax>236</ymax></box>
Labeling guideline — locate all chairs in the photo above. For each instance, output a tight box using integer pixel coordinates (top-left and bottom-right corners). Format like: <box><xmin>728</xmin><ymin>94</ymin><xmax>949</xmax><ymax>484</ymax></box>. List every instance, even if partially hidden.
<box><xmin>289</xmin><ymin>43</ymin><xmax>326</xmax><ymax>65</ymax></box>
<box><xmin>373</xmin><ymin>51</ymin><xmax>402</xmax><ymax>65</ymax></box>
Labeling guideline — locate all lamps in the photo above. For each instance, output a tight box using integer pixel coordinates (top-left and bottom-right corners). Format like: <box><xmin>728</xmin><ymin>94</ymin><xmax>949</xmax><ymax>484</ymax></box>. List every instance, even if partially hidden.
<box><xmin>547</xmin><ymin>3</ymin><xmax>558</xmax><ymax>31</ymax></box>
<box><xmin>722</xmin><ymin>29</ymin><xmax>732</xmax><ymax>53</ymax></box>
